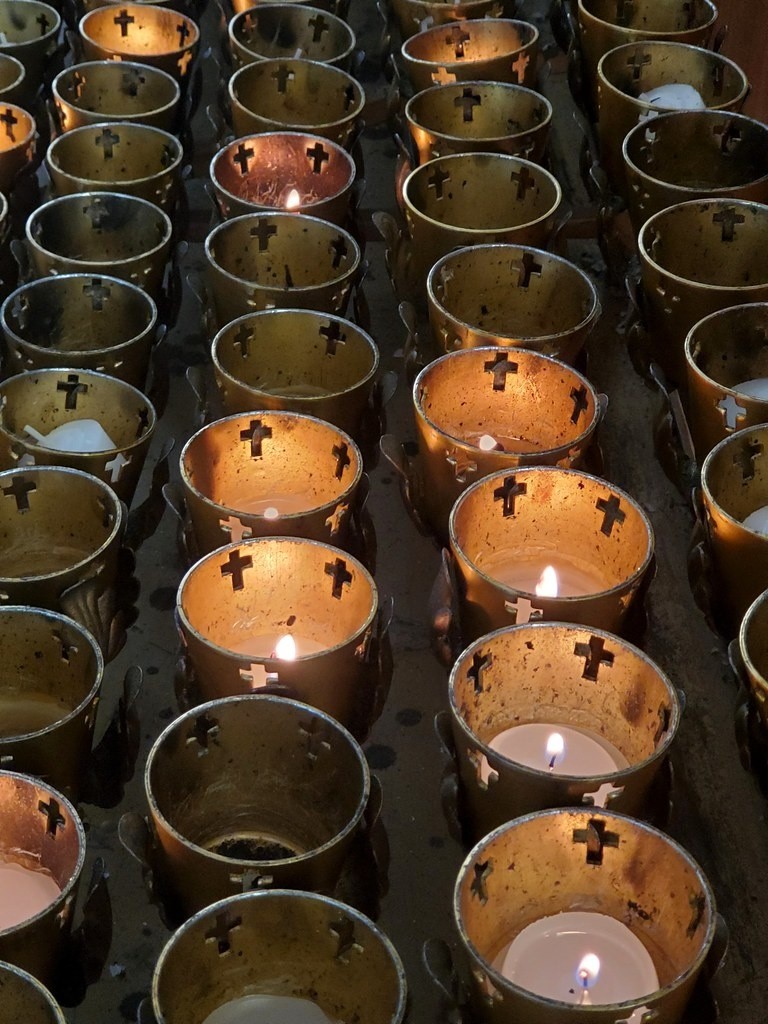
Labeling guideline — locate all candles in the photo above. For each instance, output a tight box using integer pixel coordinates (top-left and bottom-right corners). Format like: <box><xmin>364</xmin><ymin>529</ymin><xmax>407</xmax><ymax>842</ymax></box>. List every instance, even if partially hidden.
<box><xmin>1</xmin><ymin>0</ymin><xmax>768</xmax><ymax>1023</ymax></box>
